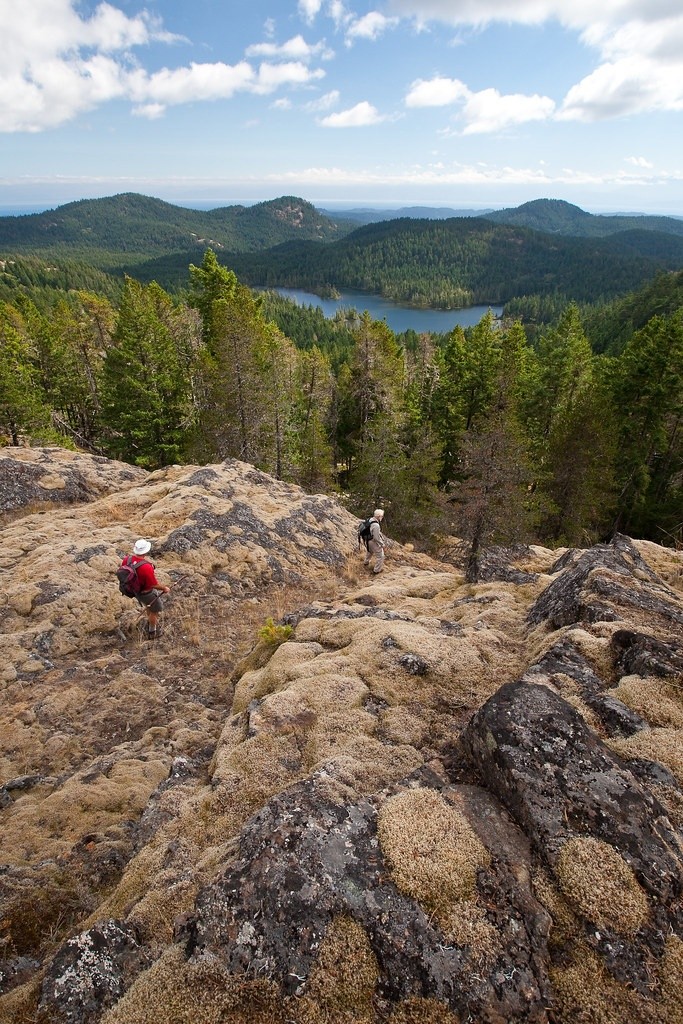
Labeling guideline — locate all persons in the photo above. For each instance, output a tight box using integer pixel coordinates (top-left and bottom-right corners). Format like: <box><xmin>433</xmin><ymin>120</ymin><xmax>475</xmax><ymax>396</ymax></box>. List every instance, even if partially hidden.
<box><xmin>120</xmin><ymin>539</ymin><xmax>170</xmax><ymax>640</ymax></box>
<box><xmin>364</xmin><ymin>509</ymin><xmax>388</xmax><ymax>575</ymax></box>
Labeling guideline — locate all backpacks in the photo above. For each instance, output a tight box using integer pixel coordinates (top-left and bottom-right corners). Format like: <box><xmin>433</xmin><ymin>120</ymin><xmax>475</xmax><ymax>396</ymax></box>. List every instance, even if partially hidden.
<box><xmin>358</xmin><ymin>517</ymin><xmax>378</xmax><ymax>552</ymax></box>
<box><xmin>117</xmin><ymin>555</ymin><xmax>147</xmax><ymax>598</ymax></box>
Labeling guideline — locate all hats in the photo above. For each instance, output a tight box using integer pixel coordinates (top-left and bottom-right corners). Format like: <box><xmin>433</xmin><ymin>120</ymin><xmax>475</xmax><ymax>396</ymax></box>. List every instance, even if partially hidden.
<box><xmin>133</xmin><ymin>539</ymin><xmax>151</xmax><ymax>555</ymax></box>
